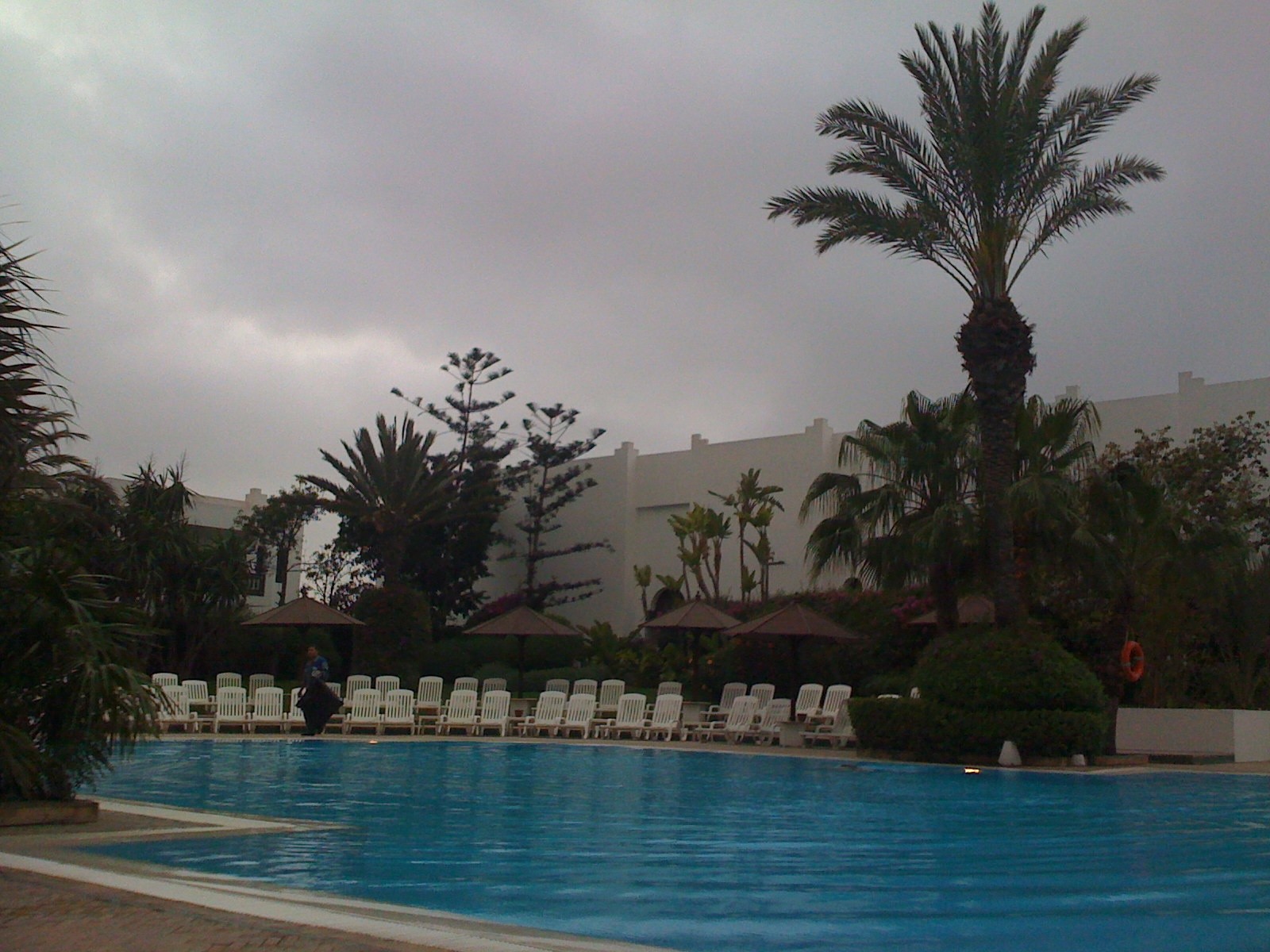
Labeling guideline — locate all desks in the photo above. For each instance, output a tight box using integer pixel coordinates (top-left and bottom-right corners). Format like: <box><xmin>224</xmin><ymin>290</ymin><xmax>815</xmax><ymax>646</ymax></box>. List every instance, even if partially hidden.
<box><xmin>509</xmin><ymin>697</ymin><xmax>538</xmax><ymax>718</ymax></box>
<box><xmin>681</xmin><ymin>700</ymin><xmax>711</xmax><ymax>729</ymax></box>
<box><xmin>774</xmin><ymin>720</ymin><xmax>809</xmax><ymax>749</ymax></box>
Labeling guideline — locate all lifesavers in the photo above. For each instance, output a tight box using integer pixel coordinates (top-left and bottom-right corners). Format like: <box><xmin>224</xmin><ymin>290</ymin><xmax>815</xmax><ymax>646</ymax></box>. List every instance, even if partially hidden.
<box><xmin>1120</xmin><ymin>641</ymin><xmax>1146</xmax><ymax>682</ymax></box>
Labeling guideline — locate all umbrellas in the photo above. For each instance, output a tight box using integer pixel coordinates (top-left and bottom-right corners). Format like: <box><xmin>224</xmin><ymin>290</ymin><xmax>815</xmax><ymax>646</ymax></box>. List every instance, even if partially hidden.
<box><xmin>905</xmin><ymin>587</ymin><xmax>1045</xmax><ymax>646</ymax></box>
<box><xmin>637</xmin><ymin>590</ymin><xmax>745</xmax><ymax>701</ymax></box>
<box><xmin>240</xmin><ymin>595</ymin><xmax>367</xmax><ymax>643</ymax></box>
<box><xmin>461</xmin><ymin>603</ymin><xmax>580</xmax><ymax>697</ymax></box>
<box><xmin>719</xmin><ymin>597</ymin><xmax>864</xmax><ymax>707</ymax></box>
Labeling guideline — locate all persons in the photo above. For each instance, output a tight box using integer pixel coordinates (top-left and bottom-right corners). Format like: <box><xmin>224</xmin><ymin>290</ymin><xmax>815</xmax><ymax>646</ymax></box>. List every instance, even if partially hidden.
<box><xmin>297</xmin><ymin>642</ymin><xmax>333</xmax><ymax>736</ymax></box>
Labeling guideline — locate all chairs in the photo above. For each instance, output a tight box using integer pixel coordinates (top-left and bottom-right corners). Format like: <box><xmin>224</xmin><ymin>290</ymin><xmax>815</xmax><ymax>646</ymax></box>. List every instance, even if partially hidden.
<box><xmin>518</xmin><ymin>678</ymin><xmax>687</xmax><ymax>742</ymax></box>
<box><xmin>104</xmin><ymin>671</ymin><xmax>309</xmax><ymax>733</ymax></box>
<box><xmin>695</xmin><ymin>681</ymin><xmax>919</xmax><ymax>751</ymax></box>
<box><xmin>324</xmin><ymin>675</ymin><xmax>512</xmax><ymax>736</ymax></box>
